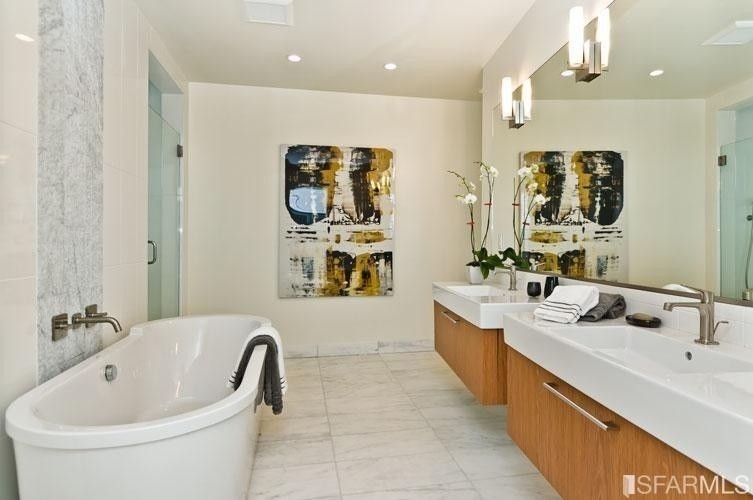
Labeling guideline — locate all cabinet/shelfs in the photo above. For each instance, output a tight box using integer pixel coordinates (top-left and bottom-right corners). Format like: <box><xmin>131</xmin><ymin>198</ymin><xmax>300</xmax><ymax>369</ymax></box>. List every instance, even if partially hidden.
<box><xmin>435</xmin><ymin>301</ymin><xmax>506</xmax><ymax>406</ymax></box>
<box><xmin>506</xmin><ymin>345</ymin><xmax>753</xmax><ymax>500</ymax></box>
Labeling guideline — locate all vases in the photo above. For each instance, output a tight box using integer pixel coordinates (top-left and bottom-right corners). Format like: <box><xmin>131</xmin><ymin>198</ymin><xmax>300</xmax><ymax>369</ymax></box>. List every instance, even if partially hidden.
<box><xmin>466</xmin><ymin>262</ymin><xmax>483</xmax><ymax>284</ymax></box>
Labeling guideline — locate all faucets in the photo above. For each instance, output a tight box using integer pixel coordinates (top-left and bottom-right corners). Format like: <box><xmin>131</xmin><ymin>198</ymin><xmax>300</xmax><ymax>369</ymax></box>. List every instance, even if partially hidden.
<box><xmin>72</xmin><ymin>313</ymin><xmax>123</xmax><ymax>334</ymax></box>
<box><xmin>662</xmin><ymin>283</ymin><xmax>730</xmax><ymax>344</ymax></box>
<box><xmin>493</xmin><ymin>265</ymin><xmax>517</xmax><ymax>291</ymax></box>
<box><xmin>531</xmin><ymin>262</ymin><xmax>547</xmax><ymax>271</ymax></box>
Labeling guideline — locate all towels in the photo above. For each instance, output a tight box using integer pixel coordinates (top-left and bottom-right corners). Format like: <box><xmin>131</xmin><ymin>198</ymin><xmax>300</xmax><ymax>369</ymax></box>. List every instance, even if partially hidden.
<box><xmin>632</xmin><ymin>313</ymin><xmax>654</xmax><ymax>320</ymax></box>
<box><xmin>663</xmin><ymin>284</ymin><xmax>696</xmax><ymax>293</ymax></box>
<box><xmin>228</xmin><ymin>325</ymin><xmax>287</xmax><ymax>415</ymax></box>
<box><xmin>580</xmin><ymin>293</ymin><xmax>626</xmax><ymax>322</ymax></box>
<box><xmin>533</xmin><ymin>285</ymin><xmax>599</xmax><ymax>324</ymax></box>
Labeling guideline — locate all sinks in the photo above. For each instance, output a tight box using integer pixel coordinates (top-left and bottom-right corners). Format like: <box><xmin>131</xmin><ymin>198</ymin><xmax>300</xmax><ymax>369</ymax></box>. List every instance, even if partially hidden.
<box><xmin>445</xmin><ymin>284</ymin><xmax>515</xmax><ymax>297</ymax></box>
<box><xmin>542</xmin><ymin>324</ymin><xmax>753</xmax><ymax>376</ymax></box>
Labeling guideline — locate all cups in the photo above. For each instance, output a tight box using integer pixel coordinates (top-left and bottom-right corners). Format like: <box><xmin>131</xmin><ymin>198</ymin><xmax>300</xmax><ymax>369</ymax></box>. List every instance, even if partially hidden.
<box><xmin>527</xmin><ymin>282</ymin><xmax>542</xmax><ymax>297</ymax></box>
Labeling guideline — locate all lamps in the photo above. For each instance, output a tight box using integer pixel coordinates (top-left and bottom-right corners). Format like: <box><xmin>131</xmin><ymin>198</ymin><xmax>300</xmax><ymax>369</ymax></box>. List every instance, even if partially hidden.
<box><xmin>587</xmin><ymin>8</ymin><xmax>610</xmax><ymax>82</ymax></box>
<box><xmin>568</xmin><ymin>7</ymin><xmax>595</xmax><ymax>82</ymax></box>
<box><xmin>516</xmin><ymin>79</ymin><xmax>531</xmax><ymax>129</ymax></box>
<box><xmin>500</xmin><ymin>76</ymin><xmax>518</xmax><ymax>128</ymax></box>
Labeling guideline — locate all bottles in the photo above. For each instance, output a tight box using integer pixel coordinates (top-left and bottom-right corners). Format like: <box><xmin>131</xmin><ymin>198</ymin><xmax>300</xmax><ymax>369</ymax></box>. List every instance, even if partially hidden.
<box><xmin>543</xmin><ymin>277</ymin><xmax>558</xmax><ymax>298</ymax></box>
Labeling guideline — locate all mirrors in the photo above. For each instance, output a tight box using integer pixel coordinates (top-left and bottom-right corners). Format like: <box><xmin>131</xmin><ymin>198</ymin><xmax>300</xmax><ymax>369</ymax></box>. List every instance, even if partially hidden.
<box><xmin>491</xmin><ymin>0</ymin><xmax>753</xmax><ymax>307</ymax></box>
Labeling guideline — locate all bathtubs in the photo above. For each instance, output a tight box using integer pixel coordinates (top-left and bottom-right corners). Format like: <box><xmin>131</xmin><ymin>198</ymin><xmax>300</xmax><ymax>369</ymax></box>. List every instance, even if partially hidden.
<box><xmin>5</xmin><ymin>313</ymin><xmax>272</xmax><ymax>500</ymax></box>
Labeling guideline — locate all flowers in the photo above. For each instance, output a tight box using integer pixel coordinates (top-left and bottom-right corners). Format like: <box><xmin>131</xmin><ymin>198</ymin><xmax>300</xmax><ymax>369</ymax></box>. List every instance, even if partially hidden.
<box><xmin>499</xmin><ymin>161</ymin><xmax>551</xmax><ymax>270</ymax></box>
<box><xmin>446</xmin><ymin>160</ymin><xmax>504</xmax><ymax>279</ymax></box>
